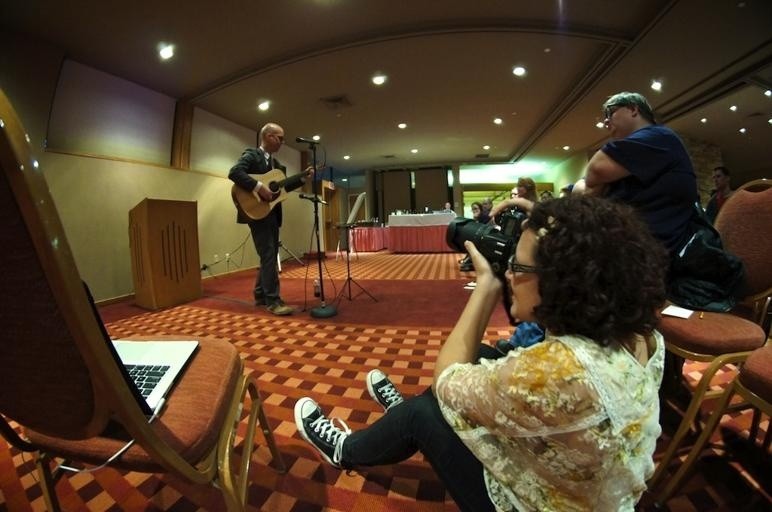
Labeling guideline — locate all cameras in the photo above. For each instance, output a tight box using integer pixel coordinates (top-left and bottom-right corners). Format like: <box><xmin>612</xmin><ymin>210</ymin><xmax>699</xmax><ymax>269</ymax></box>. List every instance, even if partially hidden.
<box><xmin>445</xmin><ymin>205</ymin><xmax>526</xmax><ymax>281</ymax></box>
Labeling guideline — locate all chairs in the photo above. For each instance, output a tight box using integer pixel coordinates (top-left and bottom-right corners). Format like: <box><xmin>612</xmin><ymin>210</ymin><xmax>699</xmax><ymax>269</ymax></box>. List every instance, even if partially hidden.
<box><xmin>655</xmin><ymin>345</ymin><xmax>772</xmax><ymax>512</ymax></box>
<box><xmin>0</xmin><ymin>91</ymin><xmax>285</xmax><ymax>511</ymax></box>
<box><xmin>647</xmin><ymin>178</ymin><xmax>771</xmax><ymax>512</ymax></box>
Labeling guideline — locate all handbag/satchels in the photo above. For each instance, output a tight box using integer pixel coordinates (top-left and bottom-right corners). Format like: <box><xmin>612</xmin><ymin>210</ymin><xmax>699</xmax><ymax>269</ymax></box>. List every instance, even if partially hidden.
<box><xmin>667</xmin><ymin>217</ymin><xmax>747</xmax><ymax>313</ymax></box>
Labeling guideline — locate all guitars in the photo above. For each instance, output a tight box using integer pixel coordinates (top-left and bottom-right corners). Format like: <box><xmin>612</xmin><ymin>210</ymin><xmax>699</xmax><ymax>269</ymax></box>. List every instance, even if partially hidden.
<box><xmin>232</xmin><ymin>161</ymin><xmax>327</xmax><ymax>224</ymax></box>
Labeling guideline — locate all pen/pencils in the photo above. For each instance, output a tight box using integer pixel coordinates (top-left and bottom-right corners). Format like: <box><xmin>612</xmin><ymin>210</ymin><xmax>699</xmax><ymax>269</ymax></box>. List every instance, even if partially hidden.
<box><xmin>699</xmin><ymin>311</ymin><xmax>704</xmax><ymax>319</ymax></box>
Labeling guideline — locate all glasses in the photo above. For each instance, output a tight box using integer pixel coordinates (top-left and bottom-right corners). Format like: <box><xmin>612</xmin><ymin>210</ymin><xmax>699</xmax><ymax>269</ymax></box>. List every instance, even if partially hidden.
<box><xmin>507</xmin><ymin>255</ymin><xmax>541</xmax><ymax>274</ymax></box>
<box><xmin>606</xmin><ymin>103</ymin><xmax>620</xmax><ymax>119</ymax></box>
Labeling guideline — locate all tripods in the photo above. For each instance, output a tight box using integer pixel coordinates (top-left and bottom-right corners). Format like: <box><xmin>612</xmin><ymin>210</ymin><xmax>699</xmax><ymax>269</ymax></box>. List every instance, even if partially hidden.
<box><xmin>337</xmin><ymin>228</ymin><xmax>378</xmax><ymax>302</ymax></box>
<box><xmin>277</xmin><ymin>234</ymin><xmax>304</xmax><ymax>272</ymax></box>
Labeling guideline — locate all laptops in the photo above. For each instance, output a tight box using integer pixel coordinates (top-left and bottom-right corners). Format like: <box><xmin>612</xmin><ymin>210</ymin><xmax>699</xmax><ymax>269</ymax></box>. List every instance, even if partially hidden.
<box><xmin>80</xmin><ymin>277</ymin><xmax>199</xmax><ymax>440</ymax></box>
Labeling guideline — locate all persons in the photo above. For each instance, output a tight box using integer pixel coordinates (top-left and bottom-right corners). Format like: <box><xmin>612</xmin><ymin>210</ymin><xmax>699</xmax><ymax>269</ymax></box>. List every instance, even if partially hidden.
<box><xmin>442</xmin><ymin>177</ymin><xmax>572</xmax><ymax>291</ymax></box>
<box><xmin>705</xmin><ymin>166</ymin><xmax>734</xmax><ymax>224</ymax></box>
<box><xmin>293</xmin><ymin>190</ymin><xmax>669</xmax><ymax>512</ymax></box>
<box><xmin>227</xmin><ymin>123</ymin><xmax>316</xmax><ymax>315</ymax></box>
<box><xmin>571</xmin><ymin>91</ymin><xmax>709</xmax><ymax>260</ymax></box>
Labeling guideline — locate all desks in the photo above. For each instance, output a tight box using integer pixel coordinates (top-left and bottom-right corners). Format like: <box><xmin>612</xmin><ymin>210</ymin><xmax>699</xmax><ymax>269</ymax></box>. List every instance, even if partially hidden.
<box><xmin>348</xmin><ymin>211</ymin><xmax>463</xmax><ymax>253</ymax></box>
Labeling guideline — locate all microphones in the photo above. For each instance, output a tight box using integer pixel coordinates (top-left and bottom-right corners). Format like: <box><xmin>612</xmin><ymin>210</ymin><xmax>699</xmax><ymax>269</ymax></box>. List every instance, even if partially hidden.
<box><xmin>299</xmin><ymin>193</ymin><xmax>329</xmax><ymax>206</ymax></box>
<box><xmin>295</xmin><ymin>136</ymin><xmax>319</xmax><ymax>145</ymax></box>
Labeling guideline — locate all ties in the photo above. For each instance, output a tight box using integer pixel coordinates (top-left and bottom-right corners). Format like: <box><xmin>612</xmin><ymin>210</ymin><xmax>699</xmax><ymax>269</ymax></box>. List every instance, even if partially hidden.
<box><xmin>267</xmin><ymin>156</ymin><xmax>272</xmax><ymax>170</ymax></box>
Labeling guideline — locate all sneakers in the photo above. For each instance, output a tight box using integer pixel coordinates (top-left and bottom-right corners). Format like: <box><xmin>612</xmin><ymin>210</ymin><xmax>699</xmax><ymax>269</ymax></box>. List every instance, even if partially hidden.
<box><xmin>294</xmin><ymin>397</ymin><xmax>352</xmax><ymax>469</ymax></box>
<box><xmin>256</xmin><ymin>300</ymin><xmax>294</xmax><ymax>315</ymax></box>
<box><xmin>366</xmin><ymin>368</ymin><xmax>404</xmax><ymax>414</ymax></box>
<box><xmin>458</xmin><ymin>255</ymin><xmax>475</xmax><ymax>272</ymax></box>
<box><xmin>496</xmin><ymin>339</ymin><xmax>513</xmax><ymax>356</ymax></box>
